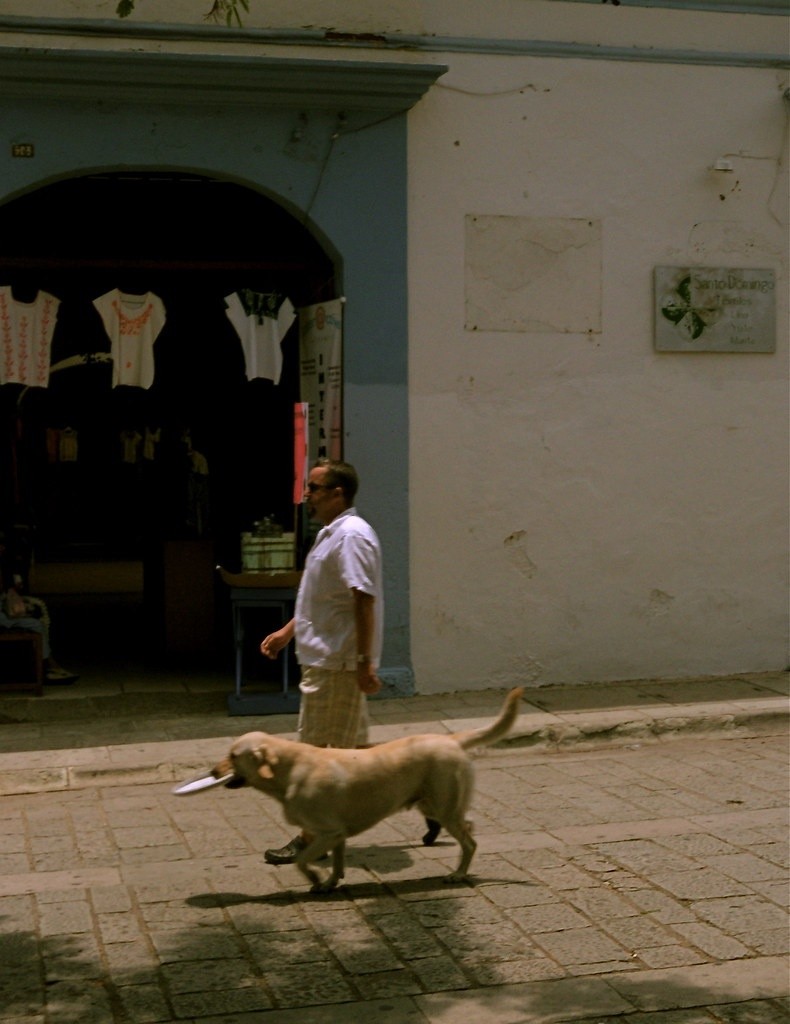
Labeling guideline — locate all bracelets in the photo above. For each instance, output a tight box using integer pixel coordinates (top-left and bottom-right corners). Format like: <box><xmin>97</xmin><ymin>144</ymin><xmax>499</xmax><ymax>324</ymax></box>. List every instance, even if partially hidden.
<box><xmin>354</xmin><ymin>652</ymin><xmax>375</xmax><ymax>665</ymax></box>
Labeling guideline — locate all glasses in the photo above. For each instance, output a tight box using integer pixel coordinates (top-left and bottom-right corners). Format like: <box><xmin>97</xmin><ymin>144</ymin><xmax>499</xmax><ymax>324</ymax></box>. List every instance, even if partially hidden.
<box><xmin>308</xmin><ymin>482</ymin><xmax>336</xmax><ymax>492</ymax></box>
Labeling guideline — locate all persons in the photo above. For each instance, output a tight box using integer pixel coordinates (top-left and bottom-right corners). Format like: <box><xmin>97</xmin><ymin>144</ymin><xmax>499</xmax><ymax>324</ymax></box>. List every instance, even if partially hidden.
<box><xmin>261</xmin><ymin>458</ymin><xmax>384</xmax><ymax>866</ymax></box>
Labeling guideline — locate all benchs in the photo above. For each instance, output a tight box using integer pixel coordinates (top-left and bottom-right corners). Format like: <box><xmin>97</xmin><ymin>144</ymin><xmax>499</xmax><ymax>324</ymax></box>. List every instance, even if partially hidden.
<box><xmin>0</xmin><ymin>625</ymin><xmax>43</xmax><ymax>695</ymax></box>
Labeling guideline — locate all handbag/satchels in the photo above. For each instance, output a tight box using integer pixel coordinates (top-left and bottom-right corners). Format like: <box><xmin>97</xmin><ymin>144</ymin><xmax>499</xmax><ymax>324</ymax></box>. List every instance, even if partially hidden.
<box><xmin>3</xmin><ymin>589</ymin><xmax>33</xmax><ymax>619</ymax></box>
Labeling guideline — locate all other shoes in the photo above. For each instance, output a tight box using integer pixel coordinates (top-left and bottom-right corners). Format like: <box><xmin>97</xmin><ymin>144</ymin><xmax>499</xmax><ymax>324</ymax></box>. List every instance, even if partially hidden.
<box><xmin>265</xmin><ymin>835</ymin><xmax>328</xmax><ymax>865</ymax></box>
<box><xmin>45</xmin><ymin>664</ymin><xmax>80</xmax><ymax>682</ymax></box>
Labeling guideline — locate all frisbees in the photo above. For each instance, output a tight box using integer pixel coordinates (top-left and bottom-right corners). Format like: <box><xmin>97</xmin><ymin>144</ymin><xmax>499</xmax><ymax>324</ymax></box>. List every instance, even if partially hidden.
<box><xmin>168</xmin><ymin>767</ymin><xmax>237</xmax><ymax>796</ymax></box>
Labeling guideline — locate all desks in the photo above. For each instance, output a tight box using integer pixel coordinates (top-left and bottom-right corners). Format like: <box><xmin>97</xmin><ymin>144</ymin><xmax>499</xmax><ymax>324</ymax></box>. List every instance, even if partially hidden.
<box><xmin>231</xmin><ymin>587</ymin><xmax>298</xmax><ymax>695</ymax></box>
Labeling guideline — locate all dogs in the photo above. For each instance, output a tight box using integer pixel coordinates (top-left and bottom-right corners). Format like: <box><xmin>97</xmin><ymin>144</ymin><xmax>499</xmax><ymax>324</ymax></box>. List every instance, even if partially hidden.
<box><xmin>211</xmin><ymin>687</ymin><xmax>526</xmax><ymax>895</ymax></box>
